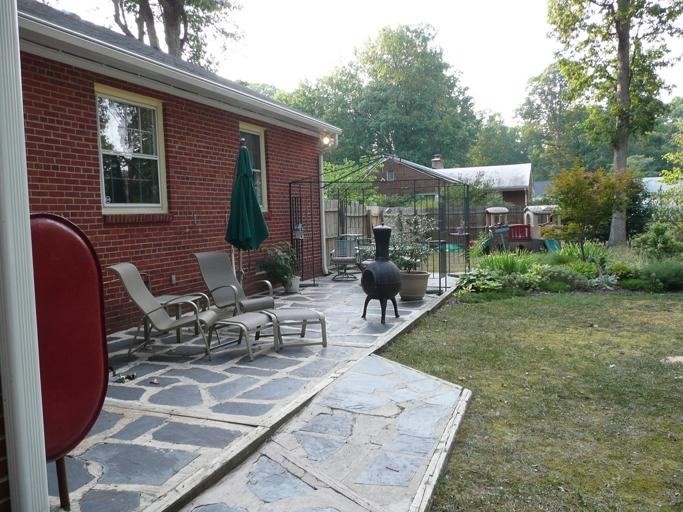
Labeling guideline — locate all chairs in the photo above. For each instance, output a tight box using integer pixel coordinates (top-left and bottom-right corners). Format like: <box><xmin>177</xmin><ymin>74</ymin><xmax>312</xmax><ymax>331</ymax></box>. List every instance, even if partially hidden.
<box><xmin>190</xmin><ymin>252</ymin><xmax>274</xmax><ymax>333</ymax></box>
<box><xmin>330</xmin><ymin>234</ymin><xmax>360</xmax><ymax>281</ymax></box>
<box><xmin>105</xmin><ymin>262</ymin><xmax>221</xmax><ymax>362</ymax></box>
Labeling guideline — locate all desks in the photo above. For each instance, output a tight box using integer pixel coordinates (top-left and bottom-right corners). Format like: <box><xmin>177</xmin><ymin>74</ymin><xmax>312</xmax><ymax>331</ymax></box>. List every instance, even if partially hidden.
<box><xmin>155</xmin><ymin>294</ymin><xmax>202</xmax><ymax>344</ymax></box>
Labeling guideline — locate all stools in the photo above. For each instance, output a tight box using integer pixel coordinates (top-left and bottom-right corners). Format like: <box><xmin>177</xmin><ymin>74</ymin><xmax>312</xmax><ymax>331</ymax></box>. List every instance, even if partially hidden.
<box><xmin>205</xmin><ymin>312</ymin><xmax>283</xmax><ymax>361</ymax></box>
<box><xmin>255</xmin><ymin>308</ymin><xmax>327</xmax><ymax>353</ymax></box>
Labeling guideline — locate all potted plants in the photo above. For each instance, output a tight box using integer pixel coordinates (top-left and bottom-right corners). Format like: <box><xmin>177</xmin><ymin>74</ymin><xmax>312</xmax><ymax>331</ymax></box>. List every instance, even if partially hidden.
<box><xmin>267</xmin><ymin>240</ymin><xmax>301</xmax><ymax>293</ymax></box>
<box><xmin>362</xmin><ymin>214</ymin><xmax>440</xmax><ymax>301</ymax></box>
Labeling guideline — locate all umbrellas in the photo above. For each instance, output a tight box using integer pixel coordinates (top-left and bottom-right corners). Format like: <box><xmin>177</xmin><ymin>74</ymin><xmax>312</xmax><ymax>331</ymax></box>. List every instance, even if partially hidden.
<box><xmin>225</xmin><ymin>138</ymin><xmax>269</xmax><ymax>287</ymax></box>
